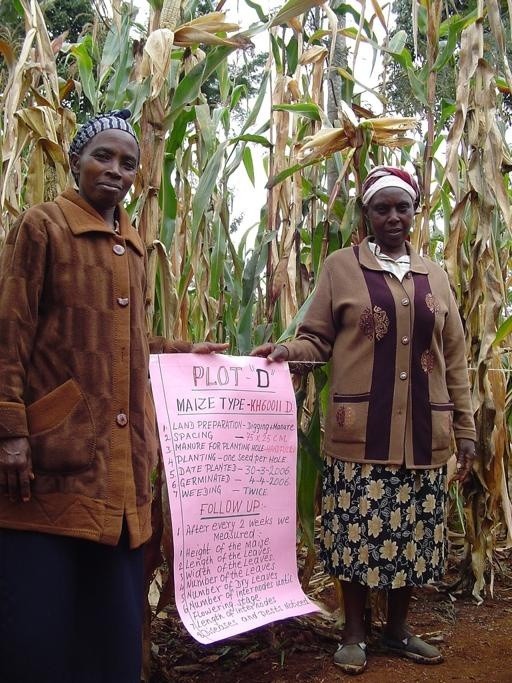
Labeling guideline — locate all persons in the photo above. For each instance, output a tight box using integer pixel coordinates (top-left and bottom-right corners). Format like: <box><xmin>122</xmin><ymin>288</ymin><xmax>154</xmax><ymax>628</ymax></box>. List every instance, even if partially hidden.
<box><xmin>252</xmin><ymin>166</ymin><xmax>478</xmax><ymax>676</ymax></box>
<box><xmin>1</xmin><ymin>108</ymin><xmax>232</xmax><ymax>680</ymax></box>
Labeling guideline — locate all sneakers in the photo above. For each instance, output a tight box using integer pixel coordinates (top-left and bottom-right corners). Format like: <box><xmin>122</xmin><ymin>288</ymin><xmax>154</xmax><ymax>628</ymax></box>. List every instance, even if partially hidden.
<box><xmin>380</xmin><ymin>630</ymin><xmax>444</xmax><ymax>664</ymax></box>
<box><xmin>334</xmin><ymin>640</ymin><xmax>368</xmax><ymax>673</ymax></box>
